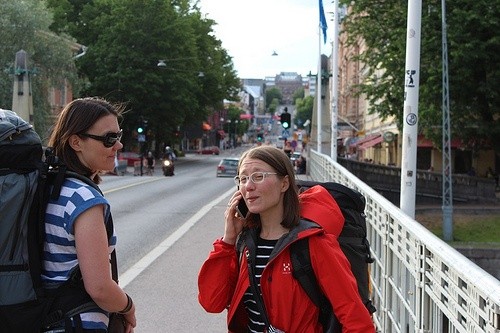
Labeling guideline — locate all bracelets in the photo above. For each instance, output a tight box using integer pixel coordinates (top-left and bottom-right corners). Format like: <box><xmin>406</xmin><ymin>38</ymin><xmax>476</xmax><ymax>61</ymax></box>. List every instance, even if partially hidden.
<box><xmin>118</xmin><ymin>294</ymin><xmax>132</xmax><ymax>313</ymax></box>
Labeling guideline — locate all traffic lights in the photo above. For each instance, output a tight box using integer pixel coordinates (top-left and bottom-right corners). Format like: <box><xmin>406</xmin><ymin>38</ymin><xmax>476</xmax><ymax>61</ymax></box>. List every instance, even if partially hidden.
<box><xmin>258</xmin><ymin>132</ymin><xmax>263</xmax><ymax>142</ymax></box>
<box><xmin>137</xmin><ymin>119</ymin><xmax>145</xmax><ymax>133</ymax></box>
<box><xmin>281</xmin><ymin>112</ymin><xmax>291</xmax><ymax>128</ymax></box>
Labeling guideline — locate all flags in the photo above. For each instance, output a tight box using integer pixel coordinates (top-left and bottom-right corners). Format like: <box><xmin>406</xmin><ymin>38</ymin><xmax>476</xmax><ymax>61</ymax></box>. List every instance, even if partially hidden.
<box><xmin>319</xmin><ymin>0</ymin><xmax>328</xmax><ymax>44</ymax></box>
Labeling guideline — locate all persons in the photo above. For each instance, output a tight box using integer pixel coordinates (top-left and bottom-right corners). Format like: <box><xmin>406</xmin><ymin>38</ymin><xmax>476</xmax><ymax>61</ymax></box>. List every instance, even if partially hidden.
<box><xmin>143</xmin><ymin>152</ymin><xmax>156</xmax><ymax>175</ymax></box>
<box><xmin>32</xmin><ymin>97</ymin><xmax>136</xmax><ymax>333</ymax></box>
<box><xmin>297</xmin><ymin>157</ymin><xmax>306</xmax><ymax>174</ymax></box>
<box><xmin>485</xmin><ymin>167</ymin><xmax>495</xmax><ymax>178</ymax></box>
<box><xmin>302</xmin><ymin>137</ymin><xmax>308</xmax><ymax>152</ymax></box>
<box><xmin>427</xmin><ymin>167</ymin><xmax>436</xmax><ymax>180</ymax></box>
<box><xmin>198</xmin><ymin>147</ymin><xmax>376</xmax><ymax>333</ymax></box>
<box><xmin>283</xmin><ymin>142</ymin><xmax>293</xmax><ymax>158</ymax></box>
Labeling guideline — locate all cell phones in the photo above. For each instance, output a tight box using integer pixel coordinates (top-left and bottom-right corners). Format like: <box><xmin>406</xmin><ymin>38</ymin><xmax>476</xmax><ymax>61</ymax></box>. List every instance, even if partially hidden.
<box><xmin>235</xmin><ymin>192</ymin><xmax>249</xmax><ymax>219</ymax></box>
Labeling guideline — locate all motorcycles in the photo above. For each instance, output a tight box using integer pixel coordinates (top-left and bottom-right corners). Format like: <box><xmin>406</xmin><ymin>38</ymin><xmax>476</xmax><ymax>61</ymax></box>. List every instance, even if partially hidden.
<box><xmin>162</xmin><ymin>154</ymin><xmax>175</xmax><ymax>176</ymax></box>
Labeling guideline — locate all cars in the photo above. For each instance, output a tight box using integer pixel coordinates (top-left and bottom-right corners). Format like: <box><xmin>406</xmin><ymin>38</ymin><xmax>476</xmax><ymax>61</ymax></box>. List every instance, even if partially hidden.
<box><xmin>196</xmin><ymin>146</ymin><xmax>220</xmax><ymax>155</ymax></box>
<box><xmin>289</xmin><ymin>152</ymin><xmax>302</xmax><ymax>166</ymax></box>
<box><xmin>216</xmin><ymin>158</ymin><xmax>239</xmax><ymax>177</ymax></box>
<box><xmin>119</xmin><ymin>151</ymin><xmax>144</xmax><ymax>166</ymax></box>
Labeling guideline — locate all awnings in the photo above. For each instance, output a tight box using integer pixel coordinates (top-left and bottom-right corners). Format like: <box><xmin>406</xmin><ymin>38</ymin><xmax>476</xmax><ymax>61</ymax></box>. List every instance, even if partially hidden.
<box><xmin>357</xmin><ymin>134</ymin><xmax>394</xmax><ymax>149</ymax></box>
<box><xmin>418</xmin><ymin>133</ymin><xmax>488</xmax><ymax>148</ymax></box>
<box><xmin>351</xmin><ymin>134</ymin><xmax>380</xmax><ymax>147</ymax></box>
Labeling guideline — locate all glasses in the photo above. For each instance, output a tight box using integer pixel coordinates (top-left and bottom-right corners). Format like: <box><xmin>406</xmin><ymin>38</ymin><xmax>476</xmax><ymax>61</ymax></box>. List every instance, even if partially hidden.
<box><xmin>234</xmin><ymin>171</ymin><xmax>279</xmax><ymax>186</ymax></box>
<box><xmin>80</xmin><ymin>129</ymin><xmax>123</xmax><ymax>148</ymax></box>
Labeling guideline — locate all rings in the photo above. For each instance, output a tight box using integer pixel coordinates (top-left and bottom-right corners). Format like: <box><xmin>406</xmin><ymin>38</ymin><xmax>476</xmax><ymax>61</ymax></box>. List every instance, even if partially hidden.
<box><xmin>228</xmin><ymin>204</ymin><xmax>232</xmax><ymax>207</ymax></box>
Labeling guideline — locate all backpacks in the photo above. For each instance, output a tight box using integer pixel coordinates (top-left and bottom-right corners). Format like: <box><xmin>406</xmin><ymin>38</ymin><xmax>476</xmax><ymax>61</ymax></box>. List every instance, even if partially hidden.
<box><xmin>0</xmin><ymin>109</ymin><xmax>125</xmax><ymax>333</ymax></box>
<box><xmin>236</xmin><ymin>178</ymin><xmax>377</xmax><ymax>333</ymax></box>
